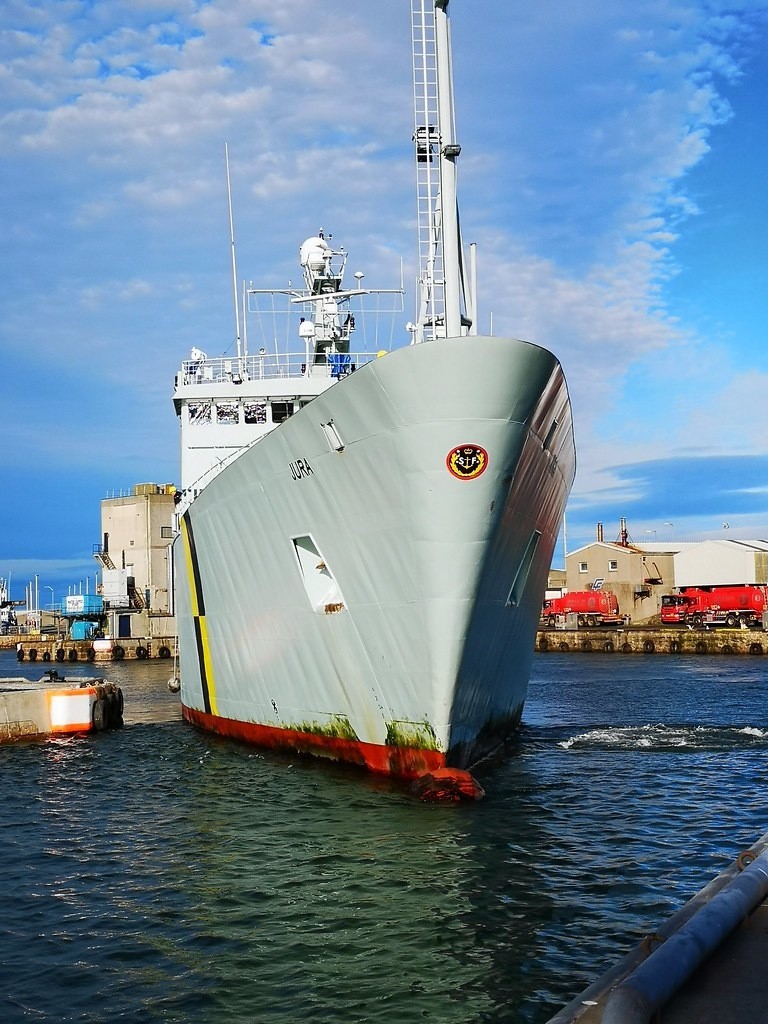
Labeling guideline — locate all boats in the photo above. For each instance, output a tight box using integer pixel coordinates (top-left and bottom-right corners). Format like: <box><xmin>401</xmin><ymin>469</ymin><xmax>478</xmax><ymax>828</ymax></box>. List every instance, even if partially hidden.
<box><xmin>164</xmin><ymin>1</ymin><xmax>578</xmax><ymax>792</ymax></box>
<box><xmin>0</xmin><ymin>669</ymin><xmax>124</xmax><ymax>744</ymax></box>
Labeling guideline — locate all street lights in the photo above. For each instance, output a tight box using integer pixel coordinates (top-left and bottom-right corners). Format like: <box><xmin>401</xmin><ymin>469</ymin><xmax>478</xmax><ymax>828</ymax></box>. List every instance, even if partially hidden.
<box><xmin>35</xmin><ymin>573</ymin><xmax>40</xmax><ymax>629</ymax></box>
<box><xmin>30</xmin><ymin>580</ymin><xmax>32</xmax><ymax>611</ymax></box>
<box><xmin>25</xmin><ymin>586</ymin><xmax>29</xmax><ymax>612</ymax></box>
<box><xmin>44</xmin><ymin>586</ymin><xmax>54</xmax><ymax>610</ymax></box>
<box><xmin>86</xmin><ymin>576</ymin><xmax>89</xmax><ymax>594</ymax></box>
<box><xmin>80</xmin><ymin>580</ymin><xmax>83</xmax><ymax>595</ymax></box>
<box><xmin>95</xmin><ymin>571</ymin><xmax>98</xmax><ymax>595</ymax></box>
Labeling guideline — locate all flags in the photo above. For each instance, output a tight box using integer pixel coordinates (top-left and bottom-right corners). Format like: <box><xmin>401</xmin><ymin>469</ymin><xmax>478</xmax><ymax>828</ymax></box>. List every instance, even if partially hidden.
<box><xmin>665</xmin><ymin>521</ymin><xmax>673</xmax><ymax>528</ymax></box>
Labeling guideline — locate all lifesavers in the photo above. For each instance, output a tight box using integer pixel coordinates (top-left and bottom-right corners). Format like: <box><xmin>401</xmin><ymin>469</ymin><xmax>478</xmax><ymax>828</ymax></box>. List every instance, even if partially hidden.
<box><xmin>94</xmin><ymin>687</ymin><xmax>124</xmax><ymax>732</ymax></box>
<box><xmin>538</xmin><ymin>637</ymin><xmax>763</xmax><ymax>656</ymax></box>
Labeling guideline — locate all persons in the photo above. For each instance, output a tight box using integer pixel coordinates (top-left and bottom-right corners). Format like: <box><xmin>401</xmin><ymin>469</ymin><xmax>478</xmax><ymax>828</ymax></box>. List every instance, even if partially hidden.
<box><xmin>169</xmin><ymin>486</ymin><xmax>183</xmax><ymax>506</ymax></box>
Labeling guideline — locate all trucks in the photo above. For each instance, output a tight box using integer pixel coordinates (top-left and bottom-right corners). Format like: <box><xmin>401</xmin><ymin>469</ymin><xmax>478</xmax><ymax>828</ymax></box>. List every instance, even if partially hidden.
<box><xmin>542</xmin><ymin>591</ymin><xmax>625</xmax><ymax>627</ymax></box>
<box><xmin>661</xmin><ymin>586</ymin><xmax>768</xmax><ymax>628</ymax></box>
